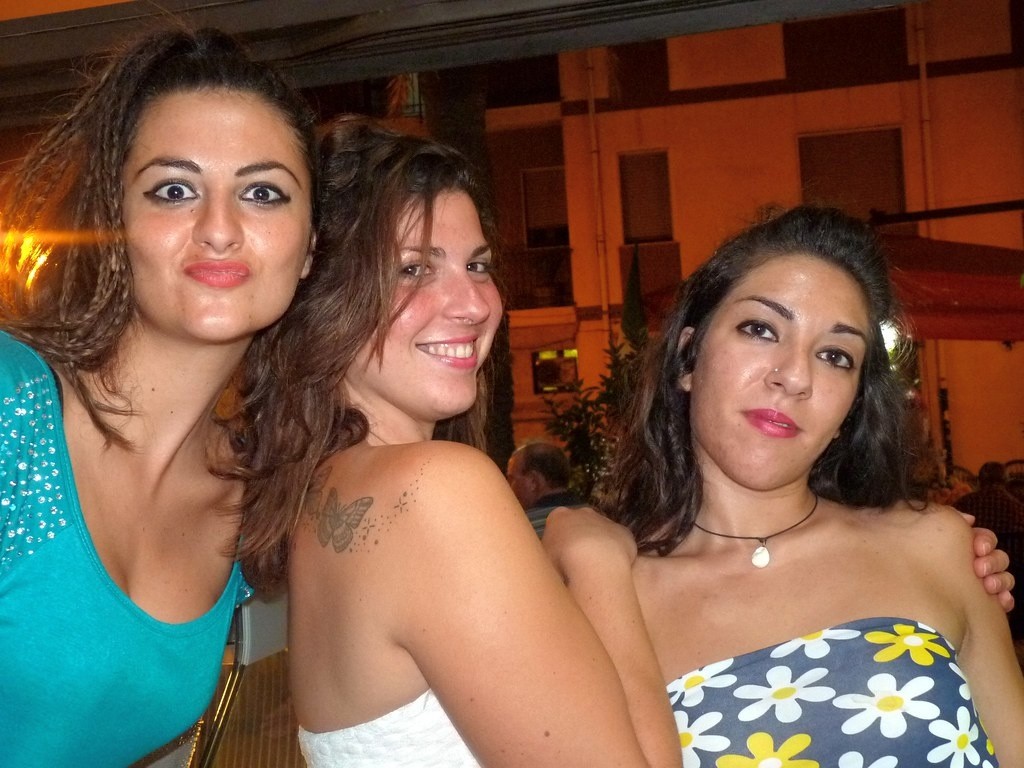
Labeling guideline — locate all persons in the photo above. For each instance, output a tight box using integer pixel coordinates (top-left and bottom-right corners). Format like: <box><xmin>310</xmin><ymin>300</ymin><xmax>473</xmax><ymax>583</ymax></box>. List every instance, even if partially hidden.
<box><xmin>201</xmin><ymin>112</ymin><xmax>1024</xmax><ymax>768</ymax></box>
<box><xmin>0</xmin><ymin>27</ymin><xmax>323</xmax><ymax>768</ymax></box>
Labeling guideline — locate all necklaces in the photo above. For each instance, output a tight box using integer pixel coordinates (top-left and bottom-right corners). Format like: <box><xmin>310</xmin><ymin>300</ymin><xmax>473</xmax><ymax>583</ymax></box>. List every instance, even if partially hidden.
<box><xmin>693</xmin><ymin>491</ymin><xmax>818</xmax><ymax>568</ymax></box>
